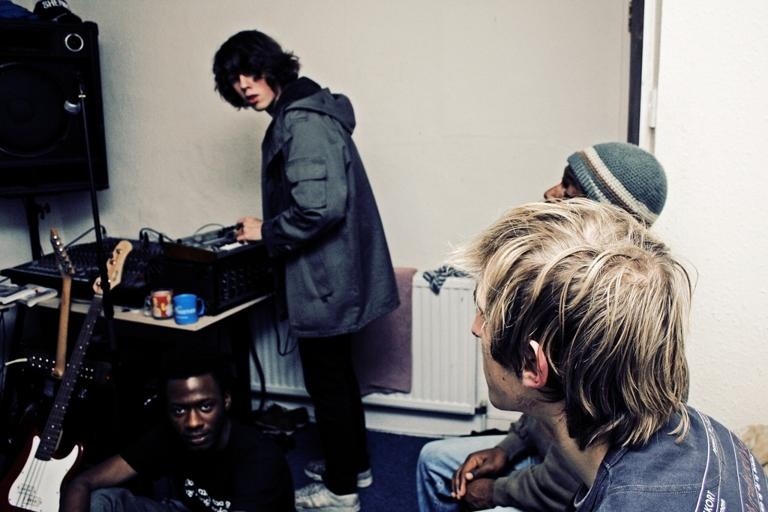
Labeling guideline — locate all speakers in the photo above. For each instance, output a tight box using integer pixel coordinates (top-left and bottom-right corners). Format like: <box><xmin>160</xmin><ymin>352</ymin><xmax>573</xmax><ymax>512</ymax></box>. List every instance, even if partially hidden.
<box><xmin>0</xmin><ymin>0</ymin><xmax>110</xmax><ymax>201</ymax></box>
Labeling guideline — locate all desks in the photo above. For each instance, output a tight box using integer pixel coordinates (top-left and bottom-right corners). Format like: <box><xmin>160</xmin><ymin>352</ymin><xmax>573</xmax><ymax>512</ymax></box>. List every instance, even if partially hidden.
<box><xmin>24</xmin><ymin>293</ymin><xmax>273</xmax><ymax>498</ymax></box>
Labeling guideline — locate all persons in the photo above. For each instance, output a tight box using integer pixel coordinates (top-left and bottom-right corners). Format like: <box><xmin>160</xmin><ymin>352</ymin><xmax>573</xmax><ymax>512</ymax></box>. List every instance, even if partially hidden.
<box><xmin>414</xmin><ymin>141</ymin><xmax>667</xmax><ymax>511</ymax></box>
<box><xmin>442</xmin><ymin>195</ymin><xmax>768</xmax><ymax>511</ymax></box>
<box><xmin>213</xmin><ymin>29</ymin><xmax>400</xmax><ymax>512</ymax></box>
<box><xmin>60</xmin><ymin>359</ymin><xmax>295</xmax><ymax>511</ymax></box>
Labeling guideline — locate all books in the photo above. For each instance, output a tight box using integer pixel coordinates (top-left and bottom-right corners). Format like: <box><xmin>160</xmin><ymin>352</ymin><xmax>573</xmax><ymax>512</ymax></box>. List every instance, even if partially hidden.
<box><xmin>0</xmin><ymin>282</ymin><xmax>58</xmax><ymax>308</ymax></box>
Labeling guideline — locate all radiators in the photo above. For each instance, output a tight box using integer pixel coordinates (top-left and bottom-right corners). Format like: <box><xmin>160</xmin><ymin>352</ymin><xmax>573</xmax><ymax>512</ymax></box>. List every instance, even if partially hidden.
<box><xmin>251</xmin><ymin>278</ymin><xmax>486</xmax><ymax>417</ymax></box>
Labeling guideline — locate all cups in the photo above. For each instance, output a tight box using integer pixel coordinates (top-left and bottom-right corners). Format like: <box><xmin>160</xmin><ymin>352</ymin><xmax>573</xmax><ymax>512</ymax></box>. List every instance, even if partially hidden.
<box><xmin>146</xmin><ymin>290</ymin><xmax>205</xmax><ymax>325</ymax></box>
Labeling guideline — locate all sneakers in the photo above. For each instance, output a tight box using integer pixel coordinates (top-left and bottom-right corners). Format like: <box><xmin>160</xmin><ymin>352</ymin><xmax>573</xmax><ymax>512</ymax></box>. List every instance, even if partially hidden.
<box><xmin>304</xmin><ymin>458</ymin><xmax>373</xmax><ymax>488</ymax></box>
<box><xmin>293</xmin><ymin>481</ymin><xmax>361</xmax><ymax>511</ymax></box>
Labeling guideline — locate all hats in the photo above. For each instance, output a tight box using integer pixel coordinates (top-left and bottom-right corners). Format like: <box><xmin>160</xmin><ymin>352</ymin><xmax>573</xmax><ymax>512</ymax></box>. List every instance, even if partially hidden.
<box><xmin>567</xmin><ymin>141</ymin><xmax>667</xmax><ymax>229</ymax></box>
